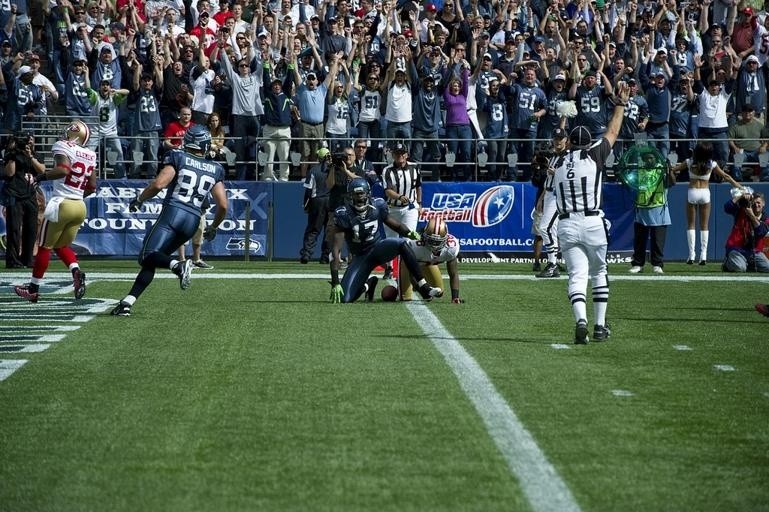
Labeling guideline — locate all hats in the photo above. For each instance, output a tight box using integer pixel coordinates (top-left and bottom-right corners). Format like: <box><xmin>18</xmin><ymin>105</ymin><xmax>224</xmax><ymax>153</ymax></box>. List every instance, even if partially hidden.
<box><xmin>394</xmin><ymin>143</ymin><xmax>408</xmax><ymax>152</ymax></box>
<box><xmin>553</xmin><ymin>125</ymin><xmax>592</xmax><ymax>149</ymax></box>
<box><xmin>743</xmin><ymin>7</ymin><xmax>752</xmax><ymax>14</ymax></box>
<box><xmin>317</xmin><ymin>148</ymin><xmax>330</xmax><ymax>158</ymax></box>
<box><xmin>711</xmin><ymin>22</ymin><xmax>721</xmax><ymax>28</ymax></box>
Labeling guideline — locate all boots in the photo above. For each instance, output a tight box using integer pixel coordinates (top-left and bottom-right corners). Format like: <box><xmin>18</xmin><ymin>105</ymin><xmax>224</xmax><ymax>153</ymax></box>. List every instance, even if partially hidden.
<box><xmin>686</xmin><ymin>229</ymin><xmax>709</xmax><ymax>265</ymax></box>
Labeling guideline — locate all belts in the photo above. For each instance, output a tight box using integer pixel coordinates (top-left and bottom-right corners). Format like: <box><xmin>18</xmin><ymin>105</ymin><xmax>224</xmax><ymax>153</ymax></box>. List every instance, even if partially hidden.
<box><xmin>301</xmin><ymin>120</ymin><xmax>322</xmax><ymax>125</ymax></box>
<box><xmin>559</xmin><ymin>211</ymin><xmax>599</xmax><ymax>220</ymax></box>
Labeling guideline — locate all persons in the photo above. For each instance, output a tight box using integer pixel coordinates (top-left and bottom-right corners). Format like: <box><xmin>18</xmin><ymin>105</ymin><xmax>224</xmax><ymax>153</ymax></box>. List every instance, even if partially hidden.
<box><xmin>326</xmin><ymin>176</ymin><xmax>443</xmax><ymax>307</ymax></box>
<box><xmin>391</xmin><ymin>217</ymin><xmax>468</xmax><ymax>304</ymax></box>
<box><xmin>0</xmin><ymin>1</ymin><xmax>767</xmax><ymax>279</ymax></box>
<box><xmin>540</xmin><ymin>81</ymin><xmax>631</xmax><ymax>346</ymax></box>
<box><xmin>109</xmin><ymin>126</ymin><xmax>228</xmax><ymax>317</ymax></box>
<box><xmin>11</xmin><ymin>119</ymin><xmax>101</xmax><ymax>302</ymax></box>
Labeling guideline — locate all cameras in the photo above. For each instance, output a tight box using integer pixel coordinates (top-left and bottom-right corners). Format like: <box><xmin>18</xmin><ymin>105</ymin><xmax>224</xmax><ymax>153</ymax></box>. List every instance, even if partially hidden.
<box><xmin>219</xmin><ymin>27</ymin><xmax>227</xmax><ymax>32</ymax></box>
<box><xmin>739</xmin><ymin>197</ymin><xmax>755</xmax><ymax>208</ymax></box>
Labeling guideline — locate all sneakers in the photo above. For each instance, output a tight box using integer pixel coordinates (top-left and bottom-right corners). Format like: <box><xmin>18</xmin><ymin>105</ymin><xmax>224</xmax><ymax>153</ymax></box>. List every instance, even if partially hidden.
<box><xmin>365</xmin><ymin>277</ymin><xmax>378</xmax><ymax>301</ymax></box>
<box><xmin>110</xmin><ymin>301</ymin><xmax>131</xmax><ymax>316</ymax></box>
<box><xmin>172</xmin><ymin>259</ymin><xmax>214</xmax><ymax>290</ymax></box>
<box><xmin>628</xmin><ymin>266</ymin><xmax>644</xmax><ymax>273</ymax></box>
<box><xmin>593</xmin><ymin>324</ymin><xmax>610</xmax><ymax>339</ymax></box>
<box><xmin>652</xmin><ymin>266</ymin><xmax>663</xmax><ymax>273</ymax></box>
<box><xmin>533</xmin><ymin>262</ymin><xmax>561</xmax><ymax>277</ymax></box>
<box><xmin>15</xmin><ymin>286</ymin><xmax>38</xmax><ymax>302</ymax></box>
<box><xmin>422</xmin><ymin>287</ymin><xmax>441</xmax><ymax>302</ymax></box>
<box><xmin>574</xmin><ymin>319</ymin><xmax>589</xmax><ymax>344</ymax></box>
<box><xmin>73</xmin><ymin>271</ymin><xmax>85</xmax><ymax>299</ymax></box>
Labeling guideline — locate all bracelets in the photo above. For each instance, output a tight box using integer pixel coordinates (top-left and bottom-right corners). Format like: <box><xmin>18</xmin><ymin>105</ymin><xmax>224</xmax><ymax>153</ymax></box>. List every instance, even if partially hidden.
<box><xmin>450</xmin><ymin>287</ymin><xmax>459</xmax><ymax>299</ymax></box>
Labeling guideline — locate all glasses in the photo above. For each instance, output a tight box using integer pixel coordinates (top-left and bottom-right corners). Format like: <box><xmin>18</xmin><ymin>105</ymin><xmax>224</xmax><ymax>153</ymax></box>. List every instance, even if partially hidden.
<box><xmin>747</xmin><ymin>61</ymin><xmax>757</xmax><ymax>64</ymax></box>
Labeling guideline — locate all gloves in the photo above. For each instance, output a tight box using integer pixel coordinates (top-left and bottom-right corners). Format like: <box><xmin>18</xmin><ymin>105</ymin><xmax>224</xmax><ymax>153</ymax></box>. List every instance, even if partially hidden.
<box><xmin>408</xmin><ymin>231</ymin><xmax>421</xmax><ymax>241</ymax></box>
<box><xmin>129</xmin><ymin>197</ymin><xmax>141</xmax><ymax>212</ymax></box>
<box><xmin>203</xmin><ymin>226</ymin><xmax>216</xmax><ymax>242</ymax></box>
<box><xmin>330</xmin><ymin>284</ymin><xmax>344</xmax><ymax>304</ymax></box>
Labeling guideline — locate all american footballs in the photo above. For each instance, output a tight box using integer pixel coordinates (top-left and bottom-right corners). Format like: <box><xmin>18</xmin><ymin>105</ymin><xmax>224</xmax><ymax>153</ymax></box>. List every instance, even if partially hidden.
<box><xmin>381</xmin><ymin>286</ymin><xmax>398</xmax><ymax>301</ymax></box>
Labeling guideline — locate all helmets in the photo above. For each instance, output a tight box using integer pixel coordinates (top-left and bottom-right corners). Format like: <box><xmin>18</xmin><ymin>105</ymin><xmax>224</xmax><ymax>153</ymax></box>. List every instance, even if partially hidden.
<box><xmin>63</xmin><ymin>120</ymin><xmax>90</xmax><ymax>146</ymax></box>
<box><xmin>182</xmin><ymin>125</ymin><xmax>211</xmax><ymax>154</ymax></box>
<box><xmin>347</xmin><ymin>179</ymin><xmax>371</xmax><ymax>211</ymax></box>
<box><xmin>422</xmin><ymin>219</ymin><xmax>448</xmax><ymax>251</ymax></box>
